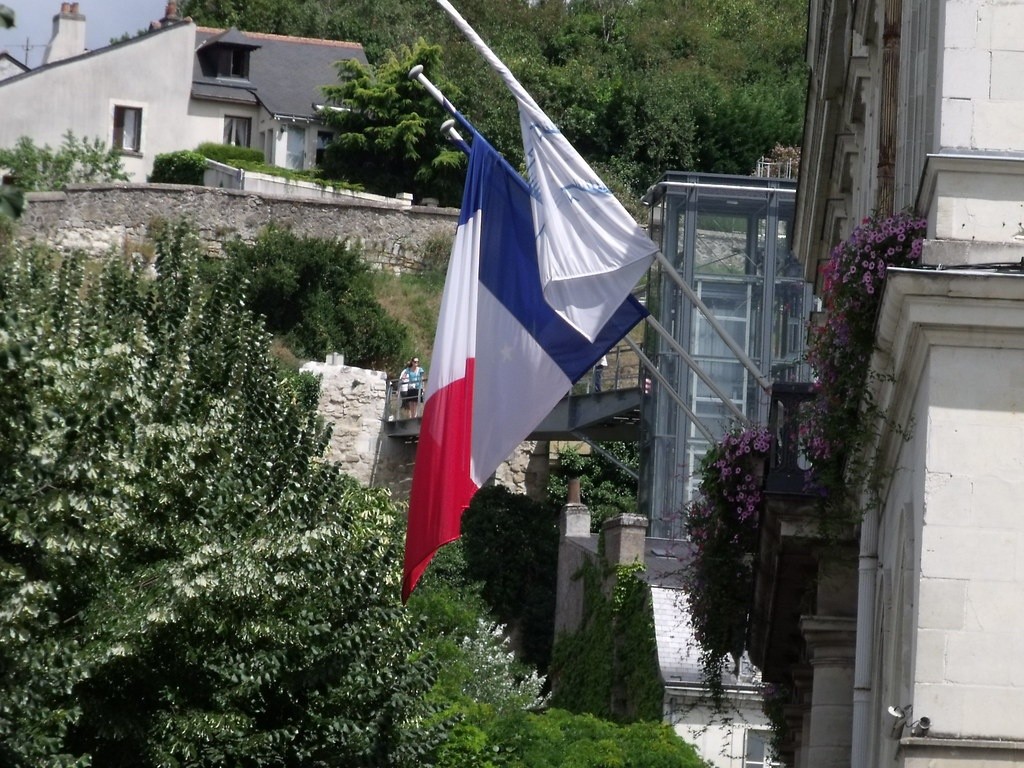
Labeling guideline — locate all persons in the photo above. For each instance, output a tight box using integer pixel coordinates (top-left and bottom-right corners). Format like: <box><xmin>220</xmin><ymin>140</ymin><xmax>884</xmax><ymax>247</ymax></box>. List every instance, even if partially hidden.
<box><xmin>402</xmin><ymin>357</ymin><xmax>424</xmax><ymax>418</ymax></box>
<box><xmin>398</xmin><ymin>361</ymin><xmax>410</xmax><ymax>418</ymax></box>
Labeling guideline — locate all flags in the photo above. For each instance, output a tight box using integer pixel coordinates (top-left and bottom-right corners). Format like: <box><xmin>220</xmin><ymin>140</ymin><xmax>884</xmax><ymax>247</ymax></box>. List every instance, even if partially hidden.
<box><xmin>400</xmin><ymin>129</ymin><xmax>651</xmax><ymax>602</ymax></box>
<box><xmin>441</xmin><ymin>4</ymin><xmax>660</xmax><ymax>342</ymax></box>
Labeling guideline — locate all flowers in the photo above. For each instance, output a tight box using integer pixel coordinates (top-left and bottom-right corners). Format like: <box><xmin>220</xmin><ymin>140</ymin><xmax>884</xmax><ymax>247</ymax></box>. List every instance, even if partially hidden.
<box><xmin>679</xmin><ymin>208</ymin><xmax>928</xmax><ymax>710</ymax></box>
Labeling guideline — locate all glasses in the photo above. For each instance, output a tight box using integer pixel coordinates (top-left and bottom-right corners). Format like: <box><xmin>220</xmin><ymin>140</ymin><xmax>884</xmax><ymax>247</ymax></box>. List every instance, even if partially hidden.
<box><xmin>414</xmin><ymin>361</ymin><xmax>419</xmax><ymax>363</ymax></box>
